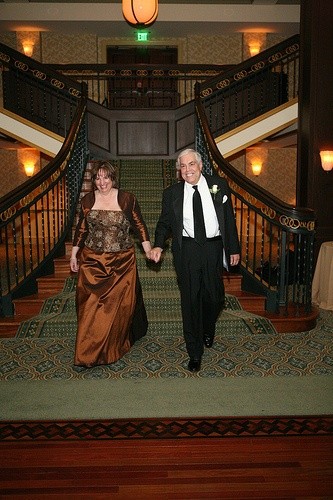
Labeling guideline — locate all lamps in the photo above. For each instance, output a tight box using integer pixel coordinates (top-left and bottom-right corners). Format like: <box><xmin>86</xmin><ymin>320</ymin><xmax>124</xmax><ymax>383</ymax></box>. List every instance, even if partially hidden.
<box><xmin>24</xmin><ymin>43</ymin><xmax>34</xmax><ymax>56</ymax></box>
<box><xmin>319</xmin><ymin>150</ymin><xmax>333</xmax><ymax>171</ymax></box>
<box><xmin>250</xmin><ymin>46</ymin><xmax>261</xmax><ymax>56</ymax></box>
<box><xmin>252</xmin><ymin>161</ymin><xmax>263</xmax><ymax>177</ymax></box>
<box><xmin>22</xmin><ymin>163</ymin><xmax>35</xmax><ymax>179</ymax></box>
<box><xmin>121</xmin><ymin>0</ymin><xmax>158</xmax><ymax>26</ymax></box>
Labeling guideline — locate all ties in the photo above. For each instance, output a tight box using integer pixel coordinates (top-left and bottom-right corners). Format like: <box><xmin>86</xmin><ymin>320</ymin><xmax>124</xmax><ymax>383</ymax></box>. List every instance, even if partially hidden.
<box><xmin>192</xmin><ymin>185</ymin><xmax>206</xmax><ymax>247</ymax></box>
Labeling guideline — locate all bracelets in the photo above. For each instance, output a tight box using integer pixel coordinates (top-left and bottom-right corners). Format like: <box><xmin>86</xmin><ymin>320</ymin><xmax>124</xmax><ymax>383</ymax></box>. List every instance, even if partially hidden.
<box><xmin>69</xmin><ymin>258</ymin><xmax>77</xmax><ymax>260</ymax></box>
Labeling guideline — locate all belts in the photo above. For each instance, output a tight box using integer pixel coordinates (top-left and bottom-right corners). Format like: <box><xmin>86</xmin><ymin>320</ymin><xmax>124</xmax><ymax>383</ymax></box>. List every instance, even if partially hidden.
<box><xmin>182</xmin><ymin>236</ymin><xmax>221</xmax><ymax>242</ymax></box>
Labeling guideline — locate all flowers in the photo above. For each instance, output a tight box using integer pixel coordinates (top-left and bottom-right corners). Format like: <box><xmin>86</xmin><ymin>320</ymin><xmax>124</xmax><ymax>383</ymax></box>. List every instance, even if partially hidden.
<box><xmin>209</xmin><ymin>184</ymin><xmax>221</xmax><ymax>200</ymax></box>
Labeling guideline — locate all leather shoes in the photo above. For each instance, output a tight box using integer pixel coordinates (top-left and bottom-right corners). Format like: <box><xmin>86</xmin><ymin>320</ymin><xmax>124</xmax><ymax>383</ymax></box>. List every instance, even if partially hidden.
<box><xmin>204</xmin><ymin>335</ymin><xmax>214</xmax><ymax>348</ymax></box>
<box><xmin>188</xmin><ymin>359</ymin><xmax>201</xmax><ymax>372</ymax></box>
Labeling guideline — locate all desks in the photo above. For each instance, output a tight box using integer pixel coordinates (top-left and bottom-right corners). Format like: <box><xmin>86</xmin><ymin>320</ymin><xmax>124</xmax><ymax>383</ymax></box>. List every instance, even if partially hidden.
<box><xmin>312</xmin><ymin>241</ymin><xmax>333</xmax><ymax>310</ymax></box>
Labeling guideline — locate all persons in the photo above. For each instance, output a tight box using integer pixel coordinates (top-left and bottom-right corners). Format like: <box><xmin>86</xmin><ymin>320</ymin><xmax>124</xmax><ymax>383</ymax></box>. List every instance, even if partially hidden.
<box><xmin>149</xmin><ymin>148</ymin><xmax>241</xmax><ymax>373</ymax></box>
<box><xmin>70</xmin><ymin>161</ymin><xmax>157</xmax><ymax>367</ymax></box>
